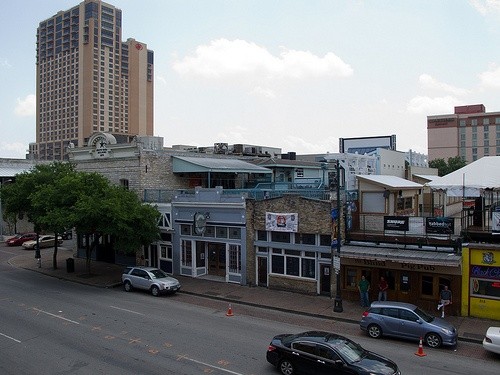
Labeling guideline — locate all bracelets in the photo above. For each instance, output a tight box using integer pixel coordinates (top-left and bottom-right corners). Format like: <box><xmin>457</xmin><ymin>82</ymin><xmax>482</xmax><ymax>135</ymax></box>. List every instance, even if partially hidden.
<box><xmin>451</xmin><ymin>301</ymin><xmax>452</xmax><ymax>302</ymax></box>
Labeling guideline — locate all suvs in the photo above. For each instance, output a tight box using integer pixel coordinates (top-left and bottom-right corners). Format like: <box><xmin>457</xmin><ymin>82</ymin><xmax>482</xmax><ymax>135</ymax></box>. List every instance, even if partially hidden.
<box><xmin>122</xmin><ymin>266</ymin><xmax>181</xmax><ymax>297</ymax></box>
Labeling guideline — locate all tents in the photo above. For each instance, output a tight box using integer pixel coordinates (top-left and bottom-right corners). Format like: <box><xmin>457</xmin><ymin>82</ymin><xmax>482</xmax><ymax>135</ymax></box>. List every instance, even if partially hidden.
<box><xmin>425</xmin><ymin>156</ymin><xmax>500</xmax><ymax>216</ymax></box>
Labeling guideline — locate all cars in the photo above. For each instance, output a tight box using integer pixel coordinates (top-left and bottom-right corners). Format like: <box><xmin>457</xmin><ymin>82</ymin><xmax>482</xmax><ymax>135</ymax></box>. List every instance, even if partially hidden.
<box><xmin>22</xmin><ymin>234</ymin><xmax>63</xmax><ymax>250</ymax></box>
<box><xmin>266</xmin><ymin>330</ymin><xmax>401</xmax><ymax>375</ymax></box>
<box><xmin>7</xmin><ymin>232</ymin><xmax>37</xmax><ymax>246</ymax></box>
<box><xmin>360</xmin><ymin>301</ymin><xmax>458</xmax><ymax>348</ymax></box>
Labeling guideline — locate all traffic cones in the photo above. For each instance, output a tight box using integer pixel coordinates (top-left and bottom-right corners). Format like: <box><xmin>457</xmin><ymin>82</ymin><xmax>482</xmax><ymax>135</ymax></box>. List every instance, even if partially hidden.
<box><xmin>225</xmin><ymin>303</ymin><xmax>234</xmax><ymax>316</ymax></box>
<box><xmin>415</xmin><ymin>336</ymin><xmax>428</xmax><ymax>357</ymax></box>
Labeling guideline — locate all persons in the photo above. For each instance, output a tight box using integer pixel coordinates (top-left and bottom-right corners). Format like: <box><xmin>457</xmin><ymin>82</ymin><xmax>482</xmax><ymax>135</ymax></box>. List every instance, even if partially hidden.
<box><xmin>378</xmin><ymin>276</ymin><xmax>388</xmax><ymax>301</ymax></box>
<box><xmin>437</xmin><ymin>286</ymin><xmax>452</xmax><ymax>319</ymax></box>
<box><xmin>358</xmin><ymin>274</ymin><xmax>370</xmax><ymax>307</ymax></box>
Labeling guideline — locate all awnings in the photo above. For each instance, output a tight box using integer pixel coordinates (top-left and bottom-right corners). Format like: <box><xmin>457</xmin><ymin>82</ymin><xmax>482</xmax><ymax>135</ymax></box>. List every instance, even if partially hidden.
<box><xmin>173</xmin><ymin>155</ymin><xmax>272</xmax><ymax>188</ymax></box>
<box><xmin>335</xmin><ymin>246</ymin><xmax>462</xmax><ymax>267</ymax></box>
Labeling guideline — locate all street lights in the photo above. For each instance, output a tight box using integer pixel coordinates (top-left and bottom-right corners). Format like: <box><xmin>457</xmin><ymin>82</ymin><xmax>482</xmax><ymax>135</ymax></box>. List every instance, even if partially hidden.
<box><xmin>331</xmin><ymin>158</ymin><xmax>343</xmax><ymax>312</ymax></box>
